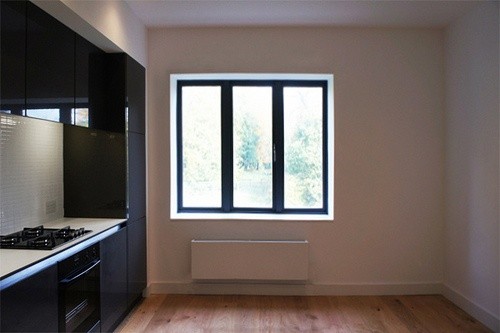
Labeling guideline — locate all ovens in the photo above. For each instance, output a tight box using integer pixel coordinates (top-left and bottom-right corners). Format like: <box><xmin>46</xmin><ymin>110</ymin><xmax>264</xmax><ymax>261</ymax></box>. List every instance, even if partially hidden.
<box><xmin>56</xmin><ymin>240</ymin><xmax>101</xmax><ymax>333</ymax></box>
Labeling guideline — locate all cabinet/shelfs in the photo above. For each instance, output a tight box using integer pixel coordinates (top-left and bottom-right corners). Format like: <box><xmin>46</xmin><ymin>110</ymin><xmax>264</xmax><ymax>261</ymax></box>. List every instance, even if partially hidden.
<box><xmin>0</xmin><ymin>219</ymin><xmax>134</xmax><ymax>333</ymax></box>
<box><xmin>94</xmin><ymin>54</ymin><xmax>150</xmax><ymax>311</ymax></box>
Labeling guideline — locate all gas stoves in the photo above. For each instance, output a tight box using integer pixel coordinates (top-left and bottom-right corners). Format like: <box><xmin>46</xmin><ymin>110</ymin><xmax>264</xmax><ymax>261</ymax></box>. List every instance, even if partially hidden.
<box><xmin>0</xmin><ymin>225</ymin><xmax>93</xmax><ymax>251</ymax></box>
